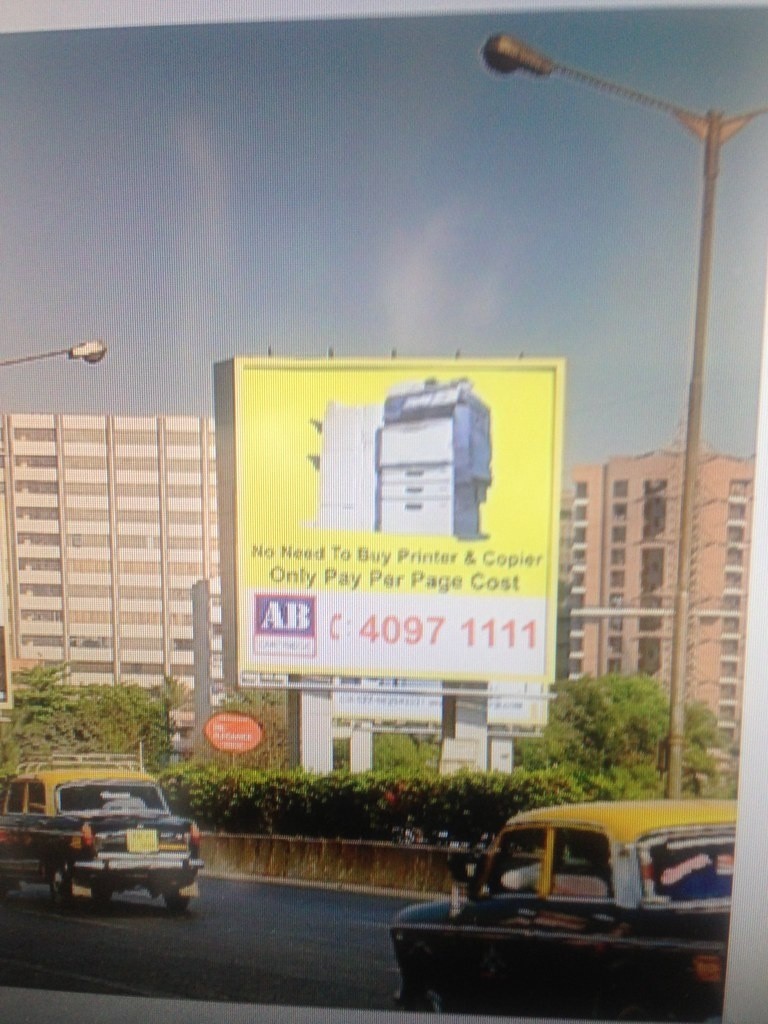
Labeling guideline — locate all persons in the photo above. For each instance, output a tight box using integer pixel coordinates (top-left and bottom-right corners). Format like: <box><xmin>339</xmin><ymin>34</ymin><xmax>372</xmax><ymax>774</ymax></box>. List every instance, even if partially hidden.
<box><xmin>499</xmin><ymin>861</ymin><xmax>542</xmax><ymax>891</ymax></box>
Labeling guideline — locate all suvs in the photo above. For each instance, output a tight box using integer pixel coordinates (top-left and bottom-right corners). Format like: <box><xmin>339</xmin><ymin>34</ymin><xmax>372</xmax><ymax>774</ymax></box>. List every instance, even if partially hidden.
<box><xmin>0</xmin><ymin>755</ymin><xmax>205</xmax><ymax>918</ymax></box>
<box><xmin>390</xmin><ymin>800</ymin><xmax>738</xmax><ymax>1024</ymax></box>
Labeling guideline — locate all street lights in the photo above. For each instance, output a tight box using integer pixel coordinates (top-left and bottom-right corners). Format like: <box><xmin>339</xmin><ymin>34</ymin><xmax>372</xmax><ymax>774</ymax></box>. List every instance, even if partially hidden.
<box><xmin>479</xmin><ymin>32</ymin><xmax>768</xmax><ymax>800</ymax></box>
<box><xmin>1</xmin><ymin>340</ymin><xmax>108</xmax><ymax>714</ymax></box>
